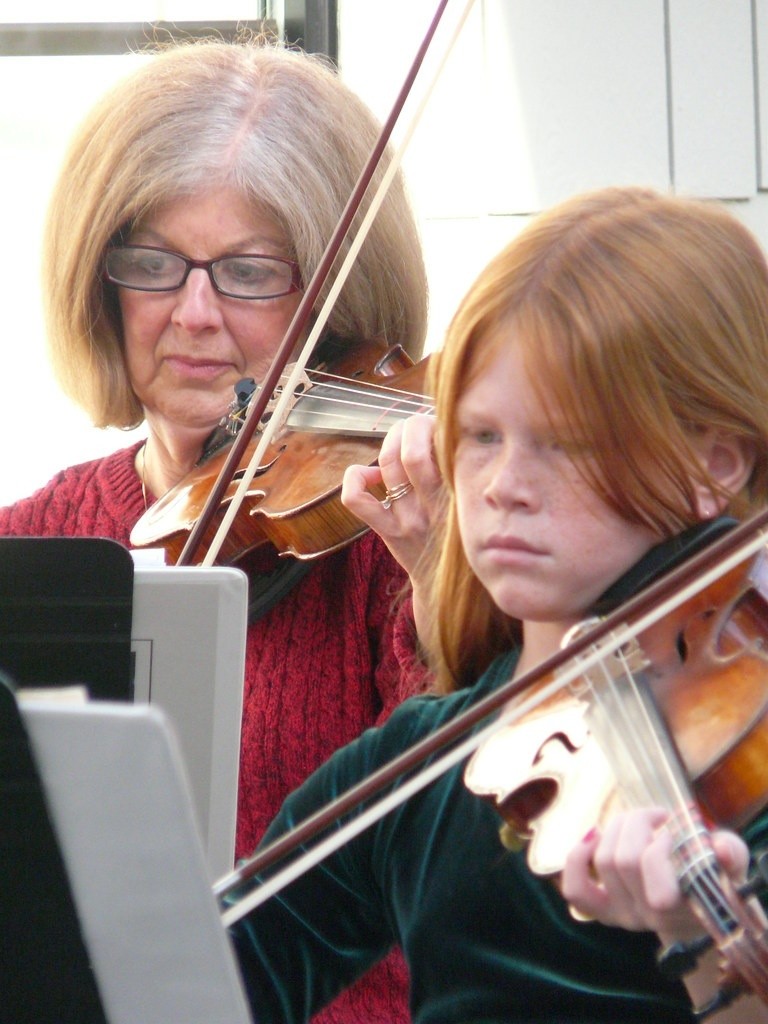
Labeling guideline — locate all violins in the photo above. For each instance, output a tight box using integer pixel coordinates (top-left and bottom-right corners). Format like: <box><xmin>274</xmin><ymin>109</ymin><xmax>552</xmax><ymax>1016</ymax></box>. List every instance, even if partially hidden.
<box><xmin>466</xmin><ymin>519</ymin><xmax>768</xmax><ymax>1024</ymax></box>
<box><xmin>129</xmin><ymin>336</ymin><xmax>444</xmax><ymax>565</ymax></box>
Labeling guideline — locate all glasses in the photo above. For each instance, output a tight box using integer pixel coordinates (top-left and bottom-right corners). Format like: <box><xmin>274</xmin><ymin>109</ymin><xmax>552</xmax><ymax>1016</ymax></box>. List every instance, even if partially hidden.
<box><xmin>103</xmin><ymin>242</ymin><xmax>303</xmax><ymax>299</ymax></box>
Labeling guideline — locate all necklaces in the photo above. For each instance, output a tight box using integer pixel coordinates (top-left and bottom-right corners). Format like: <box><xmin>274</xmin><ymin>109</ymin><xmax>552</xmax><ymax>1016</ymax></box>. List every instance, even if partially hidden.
<box><xmin>140</xmin><ymin>438</ymin><xmax>150</xmax><ymax>516</ymax></box>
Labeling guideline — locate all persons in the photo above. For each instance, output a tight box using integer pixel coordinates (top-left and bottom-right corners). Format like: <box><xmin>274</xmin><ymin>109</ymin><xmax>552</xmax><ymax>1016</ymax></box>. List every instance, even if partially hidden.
<box><xmin>216</xmin><ymin>192</ymin><xmax>768</xmax><ymax>1024</ymax></box>
<box><xmin>1</xmin><ymin>39</ymin><xmax>449</xmax><ymax>1023</ymax></box>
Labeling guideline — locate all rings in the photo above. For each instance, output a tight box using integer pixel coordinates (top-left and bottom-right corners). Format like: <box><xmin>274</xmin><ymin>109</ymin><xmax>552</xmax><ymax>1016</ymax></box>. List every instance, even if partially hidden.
<box><xmin>381</xmin><ymin>478</ymin><xmax>414</xmax><ymax>509</ymax></box>
